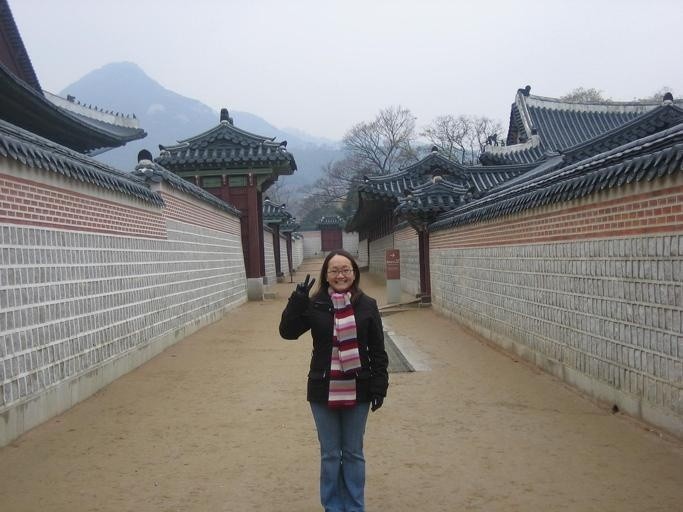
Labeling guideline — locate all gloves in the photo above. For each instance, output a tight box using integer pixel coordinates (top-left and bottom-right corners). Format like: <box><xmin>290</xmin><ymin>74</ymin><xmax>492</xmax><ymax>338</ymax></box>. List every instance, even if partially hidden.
<box><xmin>371</xmin><ymin>394</ymin><xmax>383</xmax><ymax>411</ymax></box>
<box><xmin>296</xmin><ymin>274</ymin><xmax>315</xmax><ymax>297</ymax></box>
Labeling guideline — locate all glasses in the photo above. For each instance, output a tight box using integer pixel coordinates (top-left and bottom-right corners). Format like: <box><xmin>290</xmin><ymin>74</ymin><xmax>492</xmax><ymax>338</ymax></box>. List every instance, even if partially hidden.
<box><xmin>327</xmin><ymin>268</ymin><xmax>354</xmax><ymax>274</ymax></box>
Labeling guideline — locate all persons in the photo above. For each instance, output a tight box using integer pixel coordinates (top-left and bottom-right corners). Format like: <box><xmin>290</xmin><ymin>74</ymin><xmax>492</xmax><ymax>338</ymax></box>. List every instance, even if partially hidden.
<box><xmin>279</xmin><ymin>249</ymin><xmax>389</xmax><ymax>512</ymax></box>
